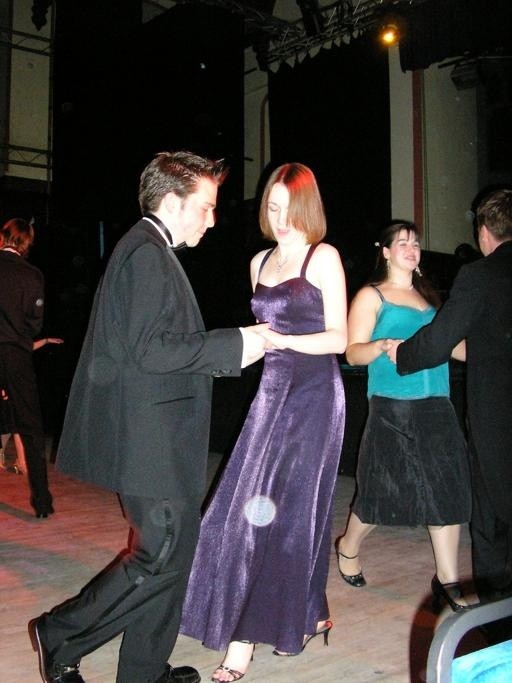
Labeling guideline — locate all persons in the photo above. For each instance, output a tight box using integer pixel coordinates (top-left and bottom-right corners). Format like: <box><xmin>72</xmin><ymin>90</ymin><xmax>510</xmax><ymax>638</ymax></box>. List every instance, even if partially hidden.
<box><xmin>385</xmin><ymin>189</ymin><xmax>512</xmax><ymax>642</ymax></box>
<box><xmin>26</xmin><ymin>154</ymin><xmax>271</xmax><ymax>682</ymax></box>
<box><xmin>177</xmin><ymin>162</ymin><xmax>348</xmax><ymax>683</ymax></box>
<box><xmin>333</xmin><ymin>220</ymin><xmax>472</xmax><ymax>614</ymax></box>
<box><xmin>0</xmin><ymin>213</ymin><xmax>65</xmax><ymax>517</ymax></box>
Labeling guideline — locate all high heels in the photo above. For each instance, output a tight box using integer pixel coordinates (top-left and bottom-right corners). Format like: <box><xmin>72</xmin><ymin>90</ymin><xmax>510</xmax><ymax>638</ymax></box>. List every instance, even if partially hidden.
<box><xmin>212</xmin><ymin>640</ymin><xmax>255</xmax><ymax>683</ymax></box>
<box><xmin>335</xmin><ymin>536</ymin><xmax>365</xmax><ymax>587</ymax></box>
<box><xmin>431</xmin><ymin>573</ymin><xmax>471</xmax><ymax>613</ymax></box>
<box><xmin>273</xmin><ymin>621</ymin><xmax>333</xmax><ymax>656</ymax></box>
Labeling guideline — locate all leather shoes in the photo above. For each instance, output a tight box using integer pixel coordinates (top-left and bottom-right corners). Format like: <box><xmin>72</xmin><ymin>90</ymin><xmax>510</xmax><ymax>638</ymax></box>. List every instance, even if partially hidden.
<box><xmin>36</xmin><ymin>506</ymin><xmax>55</xmax><ymax>519</ymax></box>
<box><xmin>28</xmin><ymin>617</ymin><xmax>85</xmax><ymax>683</ymax></box>
<box><xmin>154</xmin><ymin>664</ymin><xmax>201</xmax><ymax>683</ymax></box>
